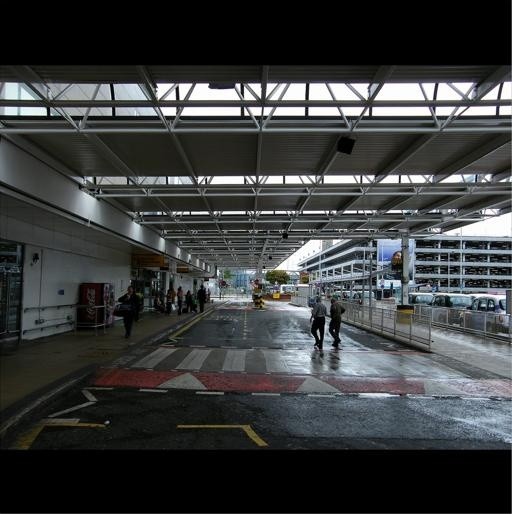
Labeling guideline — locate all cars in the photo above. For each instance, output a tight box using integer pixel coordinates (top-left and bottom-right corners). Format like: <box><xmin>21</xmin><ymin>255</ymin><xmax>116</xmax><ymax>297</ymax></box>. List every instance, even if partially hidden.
<box><xmin>332</xmin><ymin>288</ymin><xmax>377</xmax><ymax>307</ymax></box>
<box><xmin>406</xmin><ymin>290</ymin><xmax>511</xmax><ymax>335</ymax></box>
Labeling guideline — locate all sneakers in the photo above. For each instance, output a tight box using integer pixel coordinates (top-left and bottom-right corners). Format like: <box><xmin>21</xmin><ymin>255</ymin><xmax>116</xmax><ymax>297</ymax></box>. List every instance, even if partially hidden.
<box><xmin>314</xmin><ymin>339</ymin><xmax>341</xmax><ymax>348</ymax></box>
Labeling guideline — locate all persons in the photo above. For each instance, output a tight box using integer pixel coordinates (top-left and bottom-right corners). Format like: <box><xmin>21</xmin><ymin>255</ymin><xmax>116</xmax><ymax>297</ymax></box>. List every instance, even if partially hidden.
<box><xmin>113</xmin><ymin>285</ymin><xmax>141</xmax><ymax>340</ymax></box>
<box><xmin>309</xmin><ymin>296</ymin><xmax>327</xmax><ymax>350</ymax></box>
<box><xmin>152</xmin><ymin>281</ymin><xmax>211</xmax><ymax>317</ymax></box>
<box><xmin>381</xmin><ymin>251</ymin><xmax>403</xmax><ymax>280</ymax></box>
<box><xmin>328</xmin><ymin>299</ymin><xmax>347</xmax><ymax>348</ymax></box>
